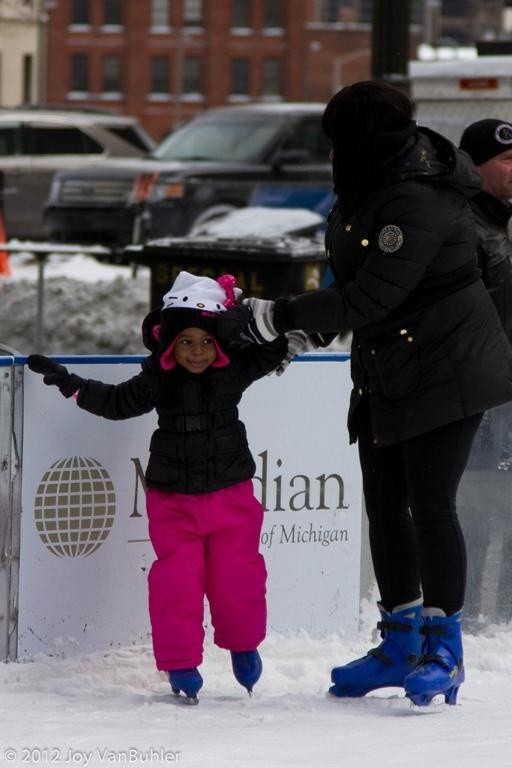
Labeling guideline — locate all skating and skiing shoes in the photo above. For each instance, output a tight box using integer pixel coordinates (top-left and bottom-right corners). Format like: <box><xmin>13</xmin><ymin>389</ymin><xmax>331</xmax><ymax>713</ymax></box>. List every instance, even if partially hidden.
<box><xmin>169</xmin><ymin>667</ymin><xmax>203</xmax><ymax>706</ymax></box>
<box><xmin>331</xmin><ymin>597</ymin><xmax>465</xmax><ymax>705</ymax></box>
<box><xmin>231</xmin><ymin>649</ymin><xmax>262</xmax><ymax>697</ymax></box>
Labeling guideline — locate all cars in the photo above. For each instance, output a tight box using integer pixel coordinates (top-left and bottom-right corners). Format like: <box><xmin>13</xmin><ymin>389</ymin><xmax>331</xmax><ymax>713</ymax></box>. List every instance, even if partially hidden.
<box><xmin>43</xmin><ymin>103</ymin><xmax>339</xmax><ymax>250</ymax></box>
<box><xmin>0</xmin><ymin>107</ymin><xmax>157</xmax><ymax>244</ymax></box>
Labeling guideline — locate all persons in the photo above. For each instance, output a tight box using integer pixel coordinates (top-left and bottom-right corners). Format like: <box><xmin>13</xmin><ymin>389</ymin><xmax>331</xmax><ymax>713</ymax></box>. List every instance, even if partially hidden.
<box><xmin>245</xmin><ymin>82</ymin><xmax>511</xmax><ymax>693</ymax></box>
<box><xmin>26</xmin><ymin>270</ymin><xmax>306</xmax><ymax>673</ymax></box>
<box><xmin>454</xmin><ymin>119</ymin><xmax>512</xmax><ymax>337</ymax></box>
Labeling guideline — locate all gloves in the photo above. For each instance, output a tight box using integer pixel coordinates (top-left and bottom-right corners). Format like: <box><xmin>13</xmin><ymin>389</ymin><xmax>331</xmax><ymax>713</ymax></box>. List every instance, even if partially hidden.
<box><xmin>242</xmin><ymin>297</ymin><xmax>321</xmax><ymax>376</ymax></box>
<box><xmin>27</xmin><ymin>354</ymin><xmax>82</xmax><ymax>399</ymax></box>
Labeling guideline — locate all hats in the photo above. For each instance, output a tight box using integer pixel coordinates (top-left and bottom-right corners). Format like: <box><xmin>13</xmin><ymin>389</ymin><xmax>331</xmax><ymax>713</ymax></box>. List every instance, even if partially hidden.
<box><xmin>459</xmin><ymin>119</ymin><xmax>512</xmax><ymax>167</ymax></box>
<box><xmin>159</xmin><ymin>270</ymin><xmax>243</xmax><ymax>341</ymax></box>
